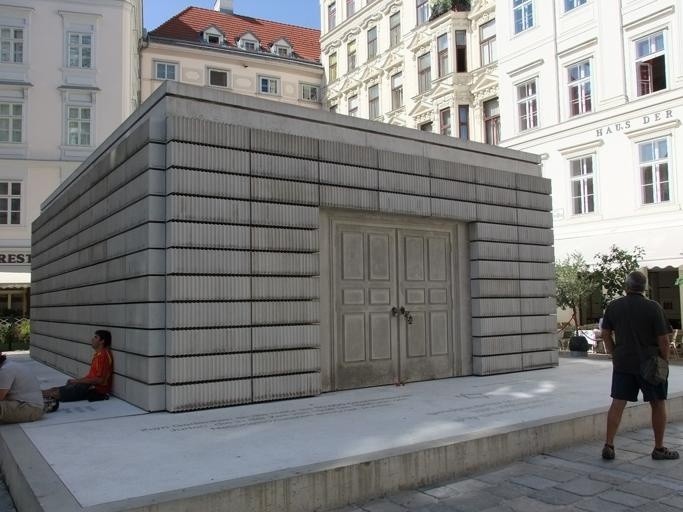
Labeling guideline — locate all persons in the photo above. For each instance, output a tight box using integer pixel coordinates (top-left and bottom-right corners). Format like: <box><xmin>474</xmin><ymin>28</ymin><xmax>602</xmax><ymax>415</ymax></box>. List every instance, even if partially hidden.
<box><xmin>41</xmin><ymin>328</ymin><xmax>115</xmax><ymax>402</ymax></box>
<box><xmin>0</xmin><ymin>350</ymin><xmax>45</xmax><ymax>424</ymax></box>
<box><xmin>600</xmin><ymin>271</ymin><xmax>679</xmax><ymax>461</ymax></box>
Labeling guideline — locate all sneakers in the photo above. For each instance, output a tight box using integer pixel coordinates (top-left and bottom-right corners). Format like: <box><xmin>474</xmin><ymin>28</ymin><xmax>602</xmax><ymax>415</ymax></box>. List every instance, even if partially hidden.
<box><xmin>651</xmin><ymin>448</ymin><xmax>678</xmax><ymax>460</ymax></box>
<box><xmin>601</xmin><ymin>446</ymin><xmax>614</xmax><ymax>460</ymax></box>
